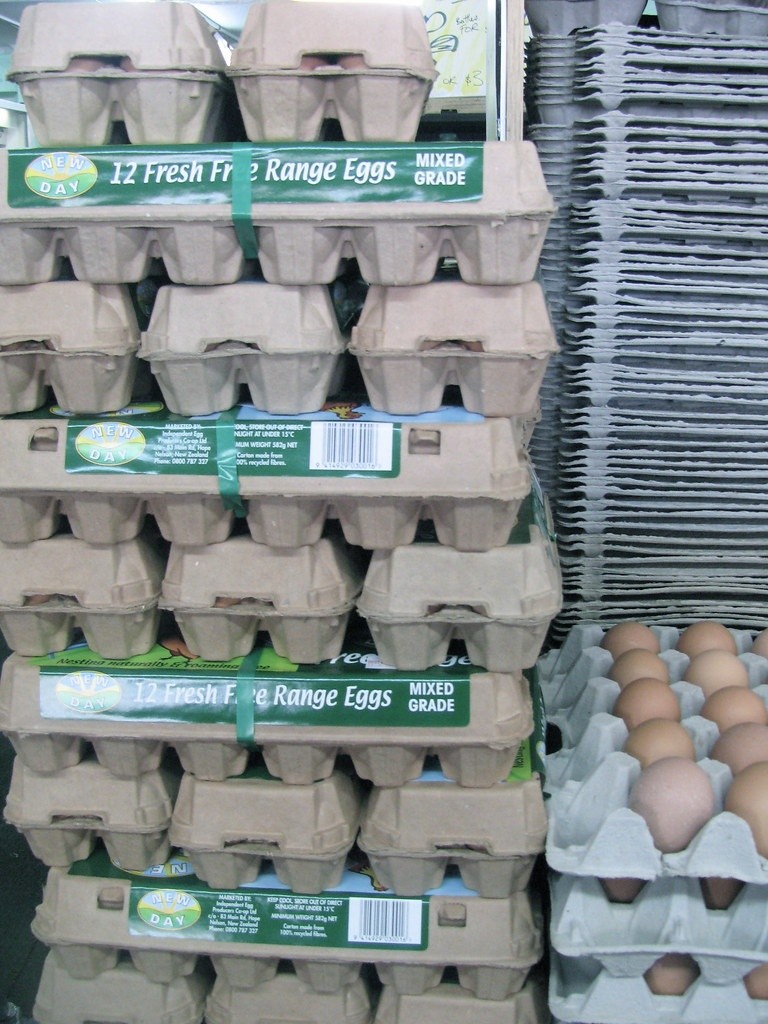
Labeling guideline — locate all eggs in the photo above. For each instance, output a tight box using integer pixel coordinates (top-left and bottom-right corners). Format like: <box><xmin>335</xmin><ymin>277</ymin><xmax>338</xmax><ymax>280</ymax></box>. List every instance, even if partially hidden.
<box><xmin>593</xmin><ymin>622</ymin><xmax>768</xmax><ymax>1003</ymax></box>
